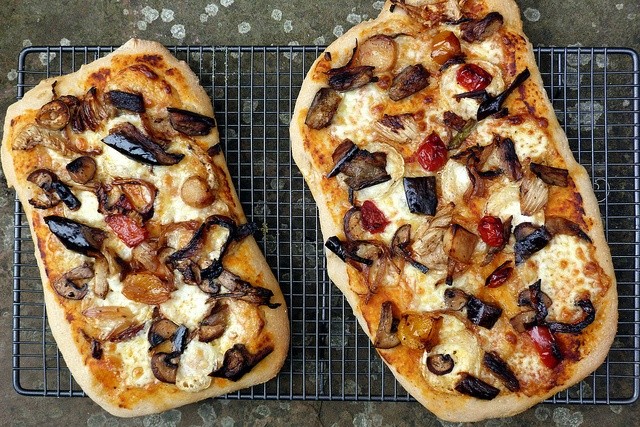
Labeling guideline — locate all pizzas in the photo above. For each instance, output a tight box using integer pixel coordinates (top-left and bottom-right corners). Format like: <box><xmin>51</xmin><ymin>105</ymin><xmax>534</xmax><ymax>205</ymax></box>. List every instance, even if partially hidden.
<box><xmin>287</xmin><ymin>0</ymin><xmax>620</xmax><ymax>425</ymax></box>
<box><xmin>1</xmin><ymin>36</ymin><xmax>291</xmax><ymax>420</ymax></box>
